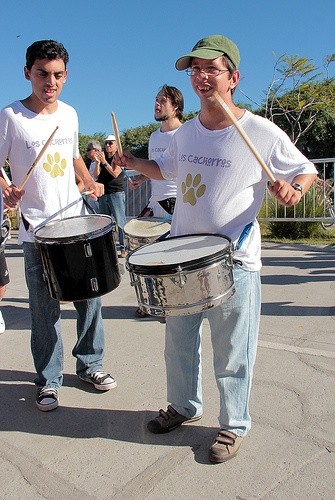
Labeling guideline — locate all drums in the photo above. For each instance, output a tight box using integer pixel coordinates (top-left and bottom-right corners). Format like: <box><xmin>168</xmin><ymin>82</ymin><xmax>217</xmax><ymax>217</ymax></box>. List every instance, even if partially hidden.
<box><xmin>123</xmin><ymin>217</ymin><xmax>238</xmax><ymax>317</ymax></box>
<box><xmin>33</xmin><ymin>214</ymin><xmax>122</xmax><ymax>302</ymax></box>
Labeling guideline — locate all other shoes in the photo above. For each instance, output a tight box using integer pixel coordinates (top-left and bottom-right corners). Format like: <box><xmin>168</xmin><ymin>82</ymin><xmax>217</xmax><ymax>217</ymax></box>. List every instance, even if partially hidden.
<box><xmin>0</xmin><ymin>310</ymin><xmax>6</xmax><ymax>334</ymax></box>
<box><xmin>121</xmin><ymin>249</ymin><xmax>126</xmax><ymax>258</ymax></box>
<box><xmin>136</xmin><ymin>308</ymin><xmax>147</xmax><ymax>318</ymax></box>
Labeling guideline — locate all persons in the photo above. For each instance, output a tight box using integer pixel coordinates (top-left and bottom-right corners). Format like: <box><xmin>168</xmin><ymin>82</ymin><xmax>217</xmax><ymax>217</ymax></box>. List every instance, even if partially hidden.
<box><xmin>0</xmin><ymin>170</ymin><xmax>10</xmax><ymax>334</ymax></box>
<box><xmin>111</xmin><ymin>34</ymin><xmax>320</xmax><ymax>463</ymax></box>
<box><xmin>75</xmin><ymin>134</ymin><xmax>129</xmax><ymax>258</ymax></box>
<box><xmin>114</xmin><ymin>83</ymin><xmax>186</xmax><ymax>323</ymax></box>
<box><xmin>0</xmin><ymin>39</ymin><xmax>118</xmax><ymax>411</ymax></box>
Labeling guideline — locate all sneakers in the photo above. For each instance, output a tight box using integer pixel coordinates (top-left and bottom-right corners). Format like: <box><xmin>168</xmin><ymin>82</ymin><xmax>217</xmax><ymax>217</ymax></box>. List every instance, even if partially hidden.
<box><xmin>79</xmin><ymin>368</ymin><xmax>117</xmax><ymax>390</ymax></box>
<box><xmin>35</xmin><ymin>387</ymin><xmax>59</xmax><ymax>411</ymax></box>
<box><xmin>208</xmin><ymin>430</ymin><xmax>246</xmax><ymax>462</ymax></box>
<box><xmin>147</xmin><ymin>405</ymin><xmax>203</xmax><ymax>433</ymax></box>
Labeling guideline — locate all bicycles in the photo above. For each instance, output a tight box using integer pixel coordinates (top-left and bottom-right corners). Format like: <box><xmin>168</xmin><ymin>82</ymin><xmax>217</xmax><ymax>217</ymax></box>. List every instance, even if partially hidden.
<box><xmin>320</xmin><ymin>172</ymin><xmax>335</xmax><ymax>230</ymax></box>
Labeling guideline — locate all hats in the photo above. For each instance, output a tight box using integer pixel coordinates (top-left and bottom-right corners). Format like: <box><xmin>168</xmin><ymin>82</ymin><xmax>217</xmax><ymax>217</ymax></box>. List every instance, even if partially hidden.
<box><xmin>105</xmin><ymin>135</ymin><xmax>116</xmax><ymax>140</ymax></box>
<box><xmin>175</xmin><ymin>35</ymin><xmax>240</xmax><ymax>71</ymax></box>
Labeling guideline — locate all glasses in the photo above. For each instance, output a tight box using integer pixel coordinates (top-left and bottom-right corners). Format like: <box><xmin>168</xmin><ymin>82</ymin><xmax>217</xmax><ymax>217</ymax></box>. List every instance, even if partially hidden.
<box><xmin>105</xmin><ymin>141</ymin><xmax>113</xmax><ymax>145</ymax></box>
<box><xmin>87</xmin><ymin>148</ymin><xmax>99</xmax><ymax>152</ymax></box>
<box><xmin>185</xmin><ymin>67</ymin><xmax>229</xmax><ymax>79</ymax></box>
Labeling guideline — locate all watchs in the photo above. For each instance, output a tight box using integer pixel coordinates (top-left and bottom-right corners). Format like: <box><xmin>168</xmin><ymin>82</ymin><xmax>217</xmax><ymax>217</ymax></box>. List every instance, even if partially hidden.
<box><xmin>291</xmin><ymin>183</ymin><xmax>303</xmax><ymax>196</ymax></box>
